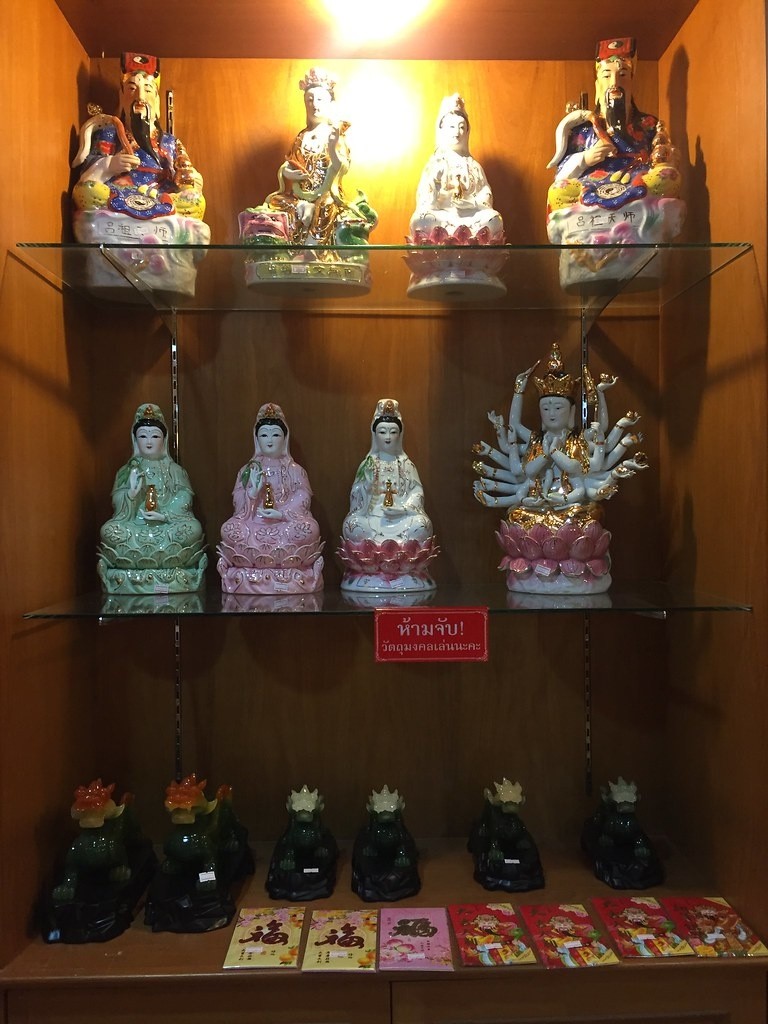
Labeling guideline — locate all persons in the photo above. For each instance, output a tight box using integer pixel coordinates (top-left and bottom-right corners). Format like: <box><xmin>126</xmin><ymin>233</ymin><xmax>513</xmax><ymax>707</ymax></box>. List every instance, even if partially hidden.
<box><xmin>469</xmin><ymin>343</ymin><xmax>650</xmax><ymax>596</ymax></box>
<box><xmin>240</xmin><ymin>67</ymin><xmax>377</xmax><ymax>296</ymax></box>
<box><xmin>546</xmin><ymin>39</ymin><xmax>688</xmax><ymax>294</ymax></box>
<box><xmin>96</xmin><ymin>403</ymin><xmax>209</xmax><ymax>595</ymax></box>
<box><xmin>70</xmin><ymin>51</ymin><xmax>212</xmax><ymax>304</ymax></box>
<box><xmin>402</xmin><ymin>92</ymin><xmax>508</xmax><ymax>300</ymax></box>
<box><xmin>334</xmin><ymin>399</ymin><xmax>440</xmax><ymax>592</ymax></box>
<box><xmin>215</xmin><ymin>403</ymin><xmax>324</xmax><ymax>595</ymax></box>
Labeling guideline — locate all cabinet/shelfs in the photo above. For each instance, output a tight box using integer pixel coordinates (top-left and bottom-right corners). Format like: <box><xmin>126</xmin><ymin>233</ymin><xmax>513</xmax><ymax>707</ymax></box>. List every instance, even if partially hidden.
<box><xmin>0</xmin><ymin>0</ymin><xmax>768</xmax><ymax>1024</ymax></box>
